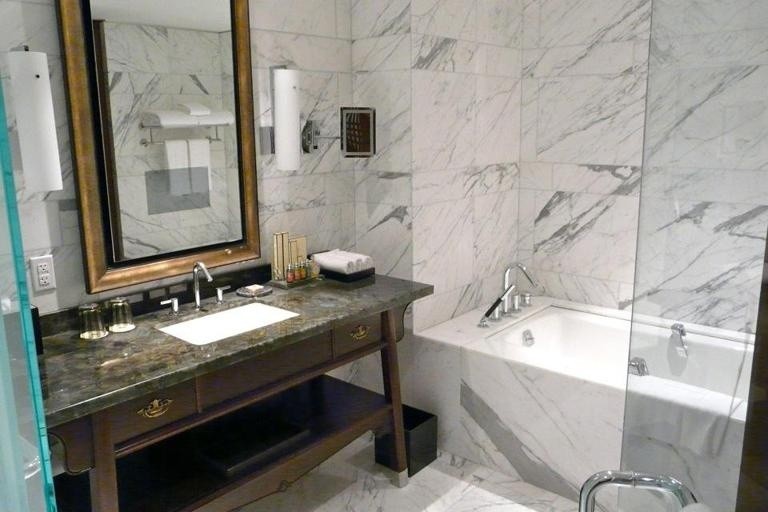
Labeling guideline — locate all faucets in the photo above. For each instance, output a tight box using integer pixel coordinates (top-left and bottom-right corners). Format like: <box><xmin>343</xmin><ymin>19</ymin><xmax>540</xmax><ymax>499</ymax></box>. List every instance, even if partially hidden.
<box><xmin>191</xmin><ymin>262</ymin><xmax>214</xmax><ymax>309</ymax></box>
<box><xmin>500</xmin><ymin>262</ymin><xmax>538</xmax><ymax>315</ymax></box>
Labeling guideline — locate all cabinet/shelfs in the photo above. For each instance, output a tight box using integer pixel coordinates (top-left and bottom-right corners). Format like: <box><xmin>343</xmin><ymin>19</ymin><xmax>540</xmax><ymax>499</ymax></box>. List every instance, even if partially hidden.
<box><xmin>86</xmin><ymin>306</ymin><xmax>410</xmax><ymax>512</ymax></box>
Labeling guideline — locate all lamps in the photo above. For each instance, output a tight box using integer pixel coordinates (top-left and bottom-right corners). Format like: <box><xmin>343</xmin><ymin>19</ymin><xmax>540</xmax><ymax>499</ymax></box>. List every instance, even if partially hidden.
<box><xmin>8</xmin><ymin>41</ymin><xmax>61</xmax><ymax>193</ymax></box>
<box><xmin>269</xmin><ymin>65</ymin><xmax>303</xmax><ymax>174</ymax></box>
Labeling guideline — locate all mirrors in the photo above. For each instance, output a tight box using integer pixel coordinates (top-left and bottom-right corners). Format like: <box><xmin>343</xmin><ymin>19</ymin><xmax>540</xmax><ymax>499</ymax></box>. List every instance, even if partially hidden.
<box><xmin>55</xmin><ymin>0</ymin><xmax>260</xmax><ymax>293</ymax></box>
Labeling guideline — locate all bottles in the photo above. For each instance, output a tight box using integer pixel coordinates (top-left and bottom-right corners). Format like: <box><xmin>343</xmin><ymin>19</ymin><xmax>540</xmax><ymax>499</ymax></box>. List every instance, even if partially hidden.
<box><xmin>286</xmin><ymin>258</ymin><xmax>312</xmax><ymax>282</ymax></box>
<box><xmin>78</xmin><ymin>302</ymin><xmax>110</xmax><ymax>340</ymax></box>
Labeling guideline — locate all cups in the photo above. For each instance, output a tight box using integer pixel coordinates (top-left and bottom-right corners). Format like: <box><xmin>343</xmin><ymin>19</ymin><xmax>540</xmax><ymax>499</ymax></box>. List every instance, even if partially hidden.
<box><xmin>310</xmin><ymin>261</ymin><xmax>320</xmax><ymax>278</ymax></box>
<box><xmin>106</xmin><ymin>297</ymin><xmax>135</xmax><ymax>334</ymax></box>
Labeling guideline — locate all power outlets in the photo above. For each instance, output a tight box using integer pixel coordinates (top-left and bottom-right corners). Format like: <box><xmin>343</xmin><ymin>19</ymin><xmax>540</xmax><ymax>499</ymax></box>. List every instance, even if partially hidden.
<box><xmin>29</xmin><ymin>254</ymin><xmax>57</xmax><ymax>294</ymax></box>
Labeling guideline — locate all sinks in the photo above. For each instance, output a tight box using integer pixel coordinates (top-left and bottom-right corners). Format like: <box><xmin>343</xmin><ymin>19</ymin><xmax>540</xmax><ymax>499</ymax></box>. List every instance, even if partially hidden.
<box><xmin>158</xmin><ymin>303</ymin><xmax>309</xmax><ymax>347</ymax></box>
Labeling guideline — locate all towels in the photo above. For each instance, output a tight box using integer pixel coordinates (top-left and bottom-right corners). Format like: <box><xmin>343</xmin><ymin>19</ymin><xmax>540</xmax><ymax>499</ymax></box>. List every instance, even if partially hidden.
<box><xmin>311</xmin><ymin>250</ymin><xmax>373</xmax><ymax>274</ymax></box>
<box><xmin>164</xmin><ymin>140</ymin><xmax>192</xmax><ymax>197</ymax></box>
<box><xmin>140</xmin><ymin>102</ymin><xmax>235</xmax><ymax>127</ymax></box>
<box><xmin>189</xmin><ymin>137</ymin><xmax>211</xmax><ymax>192</ymax></box>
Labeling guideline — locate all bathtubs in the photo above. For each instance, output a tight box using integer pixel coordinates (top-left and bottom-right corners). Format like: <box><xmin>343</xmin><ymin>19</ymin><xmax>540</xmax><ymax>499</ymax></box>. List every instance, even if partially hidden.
<box><xmin>414</xmin><ymin>295</ymin><xmax>757</xmax><ymax>512</ymax></box>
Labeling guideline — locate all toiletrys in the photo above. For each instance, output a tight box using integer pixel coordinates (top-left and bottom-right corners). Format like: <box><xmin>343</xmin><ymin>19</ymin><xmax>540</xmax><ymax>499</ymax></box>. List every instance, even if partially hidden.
<box><xmin>285</xmin><ymin>257</ymin><xmax>319</xmax><ymax>283</ymax></box>
